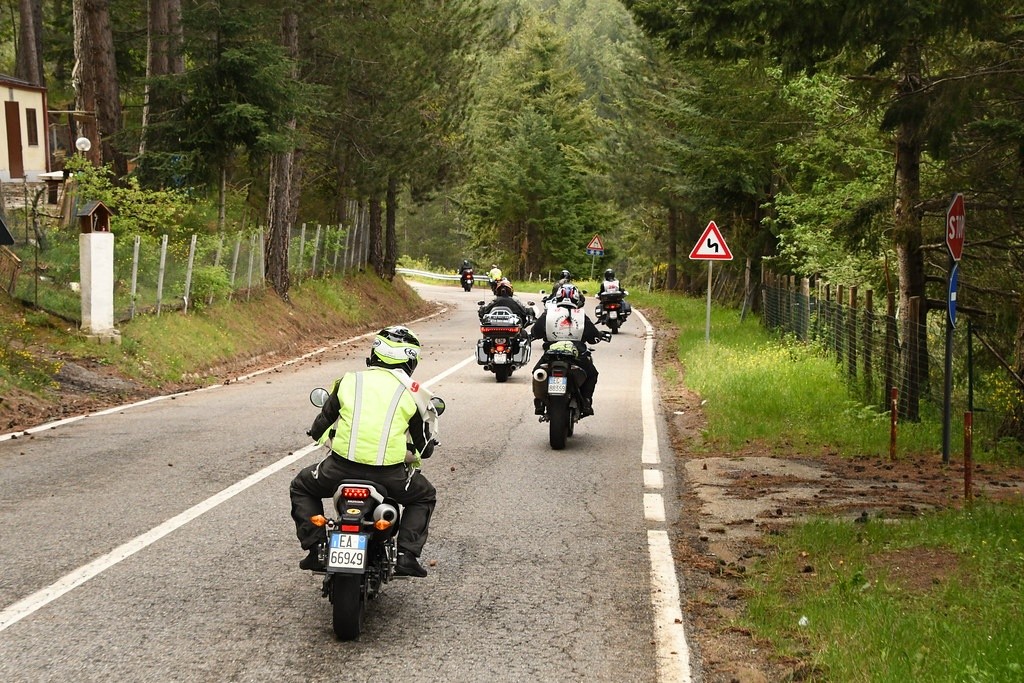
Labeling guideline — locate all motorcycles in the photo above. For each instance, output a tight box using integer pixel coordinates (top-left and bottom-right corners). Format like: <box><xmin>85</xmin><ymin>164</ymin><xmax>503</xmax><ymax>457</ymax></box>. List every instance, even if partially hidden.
<box><xmin>305</xmin><ymin>387</ymin><xmax>446</xmax><ymax>641</ymax></box>
<box><xmin>478</xmin><ymin>300</ymin><xmax>539</xmax><ymax>383</ymax></box>
<box><xmin>459</xmin><ymin>269</ymin><xmax>475</xmax><ymax>291</ymax></box>
<box><xmin>528</xmin><ymin>329</ymin><xmax>612</xmax><ymax>451</ymax></box>
<box><xmin>593</xmin><ymin>285</ymin><xmax>631</xmax><ymax>334</ymax></box>
<box><xmin>486</xmin><ymin>272</ymin><xmax>501</xmax><ymax>296</ymax></box>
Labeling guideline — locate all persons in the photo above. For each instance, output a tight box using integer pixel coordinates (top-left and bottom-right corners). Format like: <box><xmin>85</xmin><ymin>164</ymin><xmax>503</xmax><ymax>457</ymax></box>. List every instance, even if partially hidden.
<box><xmin>290</xmin><ymin>325</ymin><xmax>436</xmax><ymax>579</ymax></box>
<box><xmin>459</xmin><ymin>260</ymin><xmax>474</xmax><ymax>287</ymax></box>
<box><xmin>487</xmin><ymin>264</ymin><xmax>502</xmax><ymax>288</ymax></box>
<box><xmin>478</xmin><ymin>279</ymin><xmax>533</xmax><ymax>338</ymax></box>
<box><xmin>595</xmin><ymin>269</ymin><xmax>627</xmax><ymax>324</ymax></box>
<box><xmin>531</xmin><ymin>270</ymin><xmax>602</xmax><ymax>415</ymax></box>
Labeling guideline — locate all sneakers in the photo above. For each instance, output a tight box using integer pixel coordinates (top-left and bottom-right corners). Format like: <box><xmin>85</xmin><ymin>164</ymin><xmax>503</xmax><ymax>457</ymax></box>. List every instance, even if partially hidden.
<box><xmin>585</xmin><ymin>399</ymin><xmax>594</xmax><ymax>415</ymax></box>
<box><xmin>534</xmin><ymin>397</ymin><xmax>545</xmax><ymax>414</ymax></box>
<box><xmin>394</xmin><ymin>550</ymin><xmax>428</xmax><ymax>578</ymax></box>
<box><xmin>299</xmin><ymin>545</ymin><xmax>326</xmax><ymax>571</ymax></box>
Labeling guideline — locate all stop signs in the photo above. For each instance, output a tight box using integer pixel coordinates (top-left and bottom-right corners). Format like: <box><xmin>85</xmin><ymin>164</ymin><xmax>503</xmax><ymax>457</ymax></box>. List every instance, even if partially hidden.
<box><xmin>945</xmin><ymin>193</ymin><xmax>965</xmax><ymax>259</ymax></box>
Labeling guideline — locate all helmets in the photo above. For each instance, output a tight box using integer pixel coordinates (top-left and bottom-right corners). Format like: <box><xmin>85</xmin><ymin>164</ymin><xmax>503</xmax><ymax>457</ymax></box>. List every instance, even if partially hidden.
<box><xmin>370</xmin><ymin>326</ymin><xmax>421</xmax><ymax>376</ymax></box>
<box><xmin>463</xmin><ymin>260</ymin><xmax>468</xmax><ymax>265</ymax></box>
<box><xmin>560</xmin><ymin>270</ymin><xmax>571</xmax><ymax>282</ymax></box>
<box><xmin>495</xmin><ymin>279</ymin><xmax>514</xmax><ymax>297</ymax></box>
<box><xmin>605</xmin><ymin>269</ymin><xmax>615</xmax><ymax>280</ymax></box>
<box><xmin>556</xmin><ymin>283</ymin><xmax>581</xmax><ymax>308</ymax></box>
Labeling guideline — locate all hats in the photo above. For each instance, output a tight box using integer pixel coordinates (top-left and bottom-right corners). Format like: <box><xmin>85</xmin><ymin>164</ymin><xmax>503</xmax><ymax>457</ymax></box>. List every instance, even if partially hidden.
<box><xmin>492</xmin><ymin>264</ymin><xmax>497</xmax><ymax>268</ymax></box>
